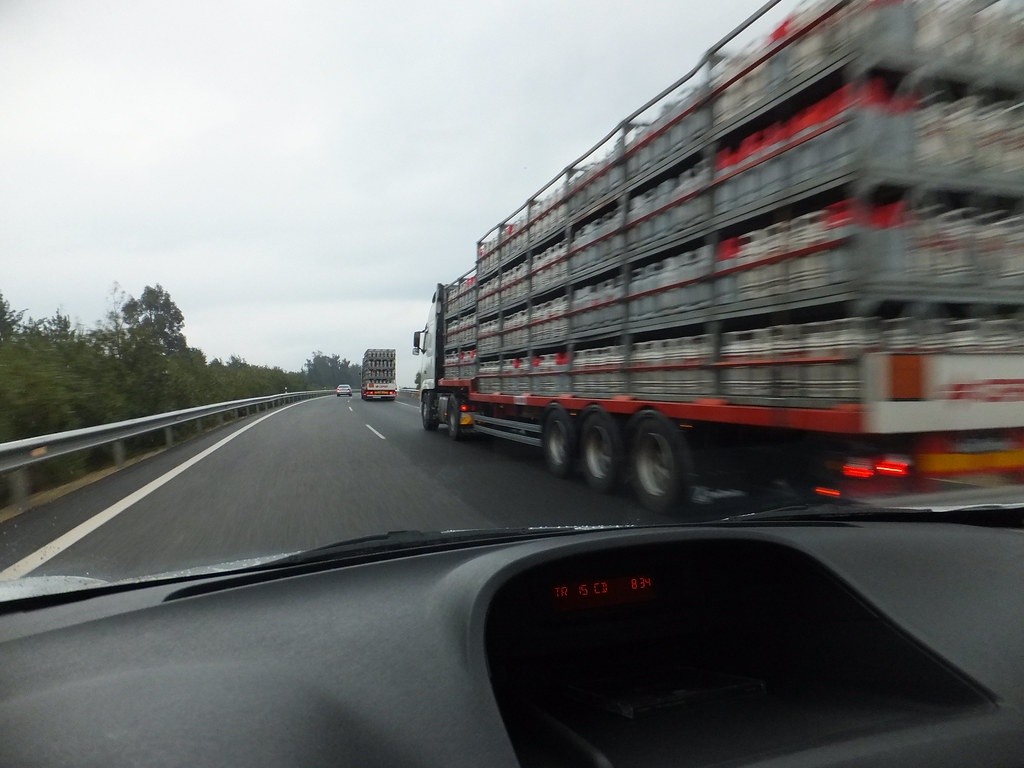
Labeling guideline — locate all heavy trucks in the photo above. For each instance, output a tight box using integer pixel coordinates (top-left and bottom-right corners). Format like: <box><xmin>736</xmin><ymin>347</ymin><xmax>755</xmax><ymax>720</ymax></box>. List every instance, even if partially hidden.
<box><xmin>359</xmin><ymin>349</ymin><xmax>398</xmax><ymax>401</ymax></box>
<box><xmin>408</xmin><ymin>0</ymin><xmax>1024</xmax><ymax>517</ymax></box>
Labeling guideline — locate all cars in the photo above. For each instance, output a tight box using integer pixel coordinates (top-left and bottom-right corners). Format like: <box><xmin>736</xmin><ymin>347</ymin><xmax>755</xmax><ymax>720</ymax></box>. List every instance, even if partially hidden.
<box><xmin>1</xmin><ymin>486</ymin><xmax>1024</xmax><ymax>768</ymax></box>
<box><xmin>336</xmin><ymin>384</ymin><xmax>352</xmax><ymax>398</ymax></box>
